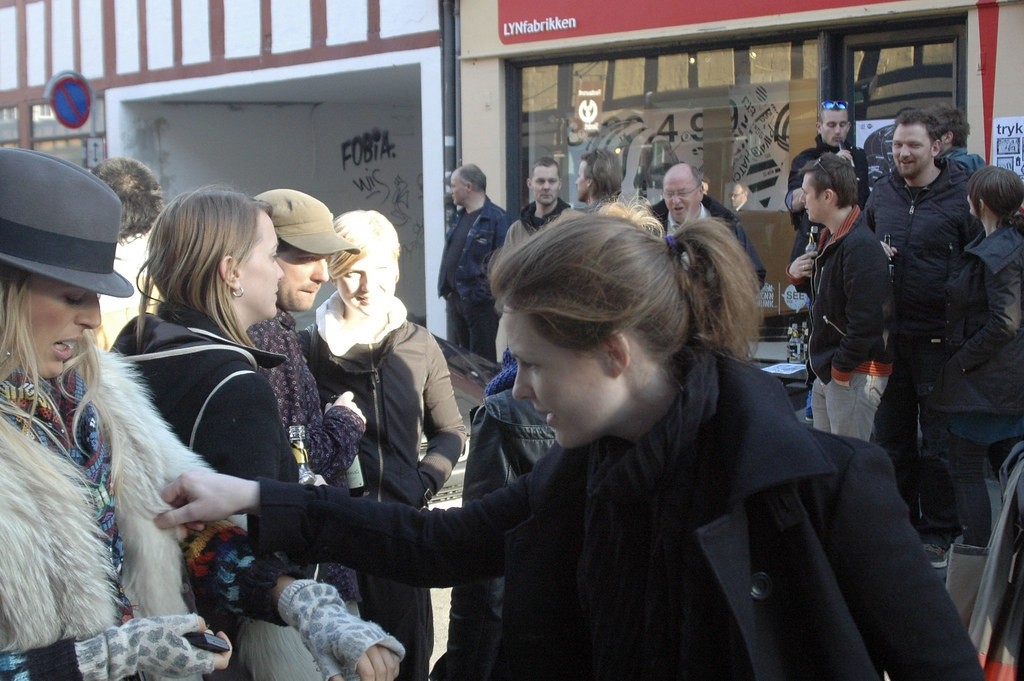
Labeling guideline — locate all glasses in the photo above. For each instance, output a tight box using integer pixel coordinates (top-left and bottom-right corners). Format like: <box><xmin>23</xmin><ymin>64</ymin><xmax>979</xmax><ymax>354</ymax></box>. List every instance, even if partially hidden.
<box><xmin>813</xmin><ymin>156</ymin><xmax>832</xmax><ymax>180</ymax></box>
<box><xmin>819</xmin><ymin>101</ymin><xmax>848</xmax><ymax>116</ymax></box>
<box><xmin>662</xmin><ymin>183</ymin><xmax>703</xmax><ymax>199</ymax></box>
<box><xmin>730</xmin><ymin>192</ymin><xmax>742</xmax><ymax>198</ymax></box>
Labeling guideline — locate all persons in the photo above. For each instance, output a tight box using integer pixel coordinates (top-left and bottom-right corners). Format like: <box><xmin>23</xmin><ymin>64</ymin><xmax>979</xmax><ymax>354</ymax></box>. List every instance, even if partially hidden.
<box><xmin>0</xmin><ymin>137</ymin><xmax>465</xmax><ymax>681</ymax></box>
<box><xmin>575</xmin><ymin>149</ymin><xmax>623</xmax><ymax>214</ymax></box>
<box><xmin>786</xmin><ymin>99</ymin><xmax>1024</xmax><ymax>569</ymax></box>
<box><xmin>437</xmin><ymin>163</ymin><xmax>515</xmax><ymax>366</ymax></box>
<box><xmin>727</xmin><ymin>181</ymin><xmax>752</xmax><ymax>211</ymax></box>
<box><xmin>649</xmin><ymin>161</ymin><xmax>767</xmax><ymax>293</ymax></box>
<box><xmin>504</xmin><ymin>157</ymin><xmax>584</xmax><ymax>249</ymax></box>
<box><xmin>157</xmin><ymin>200</ymin><xmax>988</xmax><ymax>680</ymax></box>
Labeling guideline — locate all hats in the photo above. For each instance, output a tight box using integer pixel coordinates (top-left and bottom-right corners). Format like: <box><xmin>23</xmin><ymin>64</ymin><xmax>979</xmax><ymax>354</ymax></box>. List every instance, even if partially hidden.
<box><xmin>0</xmin><ymin>146</ymin><xmax>134</xmax><ymax>297</ymax></box>
<box><xmin>253</xmin><ymin>188</ymin><xmax>360</xmax><ymax>255</ymax></box>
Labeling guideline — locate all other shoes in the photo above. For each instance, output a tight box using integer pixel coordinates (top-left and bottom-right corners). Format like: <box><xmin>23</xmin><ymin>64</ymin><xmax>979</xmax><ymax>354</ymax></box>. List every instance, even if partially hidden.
<box><xmin>922</xmin><ymin>543</ymin><xmax>947</xmax><ymax>568</ymax></box>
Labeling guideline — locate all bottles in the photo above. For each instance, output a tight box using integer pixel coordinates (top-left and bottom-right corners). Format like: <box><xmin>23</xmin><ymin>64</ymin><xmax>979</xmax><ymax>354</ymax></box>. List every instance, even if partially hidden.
<box><xmin>883</xmin><ymin>233</ymin><xmax>895</xmax><ymax>286</ymax></box>
<box><xmin>805</xmin><ymin>225</ymin><xmax>819</xmax><ymax>256</ymax></box>
<box><xmin>288</xmin><ymin>425</ymin><xmax>312</xmax><ymax>484</ymax></box>
<box><xmin>838</xmin><ymin>137</ymin><xmax>856</xmax><ymax>170</ymax></box>
<box><xmin>785</xmin><ymin>317</ymin><xmax>810</xmax><ymax>365</ymax></box>
<box><xmin>331</xmin><ymin>394</ymin><xmax>368</xmax><ymax>498</ymax></box>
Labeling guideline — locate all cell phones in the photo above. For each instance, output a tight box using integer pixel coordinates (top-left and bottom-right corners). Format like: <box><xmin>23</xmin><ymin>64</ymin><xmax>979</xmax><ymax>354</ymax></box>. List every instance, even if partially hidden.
<box><xmin>183</xmin><ymin>632</ymin><xmax>230</xmax><ymax>653</ymax></box>
